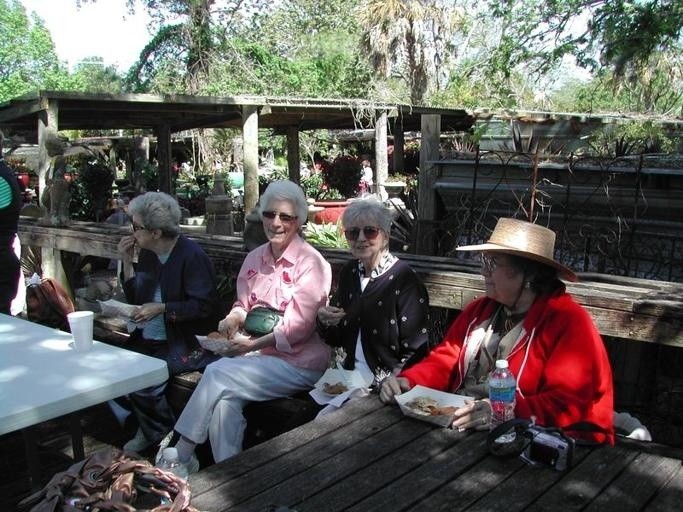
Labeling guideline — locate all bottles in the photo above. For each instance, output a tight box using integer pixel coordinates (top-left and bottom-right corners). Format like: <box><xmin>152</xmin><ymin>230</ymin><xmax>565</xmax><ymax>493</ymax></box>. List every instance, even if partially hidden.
<box><xmin>488</xmin><ymin>358</ymin><xmax>517</xmax><ymax>444</ymax></box>
<box><xmin>155</xmin><ymin>447</ymin><xmax>190</xmax><ymax>505</ymax></box>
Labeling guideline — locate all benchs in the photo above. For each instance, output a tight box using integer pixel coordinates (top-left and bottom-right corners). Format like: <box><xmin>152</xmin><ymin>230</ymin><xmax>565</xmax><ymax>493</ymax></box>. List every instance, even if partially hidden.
<box><xmin>15</xmin><ymin>227</ymin><xmax>683</xmax><ymax>454</ymax></box>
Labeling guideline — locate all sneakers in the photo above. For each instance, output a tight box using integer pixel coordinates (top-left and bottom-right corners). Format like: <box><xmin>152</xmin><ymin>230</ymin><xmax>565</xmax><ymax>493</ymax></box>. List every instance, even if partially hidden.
<box><xmin>124</xmin><ymin>428</ymin><xmax>149</xmax><ymax>451</ymax></box>
<box><xmin>154</xmin><ymin>447</ymin><xmax>199</xmax><ymax>475</ymax></box>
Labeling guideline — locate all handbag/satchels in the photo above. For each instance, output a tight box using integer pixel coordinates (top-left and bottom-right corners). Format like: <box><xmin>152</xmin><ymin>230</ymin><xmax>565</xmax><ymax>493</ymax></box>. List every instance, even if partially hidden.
<box><xmin>244</xmin><ymin>307</ymin><xmax>278</xmax><ymax>335</ymax></box>
<box><xmin>94</xmin><ymin>395</ymin><xmax>138</xmax><ymax>443</ymax></box>
<box><xmin>18</xmin><ymin>445</ymin><xmax>197</xmax><ymax>512</ymax></box>
<box><xmin>26</xmin><ymin>278</ymin><xmax>75</xmax><ymax>334</ymax></box>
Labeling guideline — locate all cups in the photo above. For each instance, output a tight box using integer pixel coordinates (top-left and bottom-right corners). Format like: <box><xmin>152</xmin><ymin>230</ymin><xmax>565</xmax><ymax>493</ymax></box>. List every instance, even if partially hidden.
<box><xmin>67</xmin><ymin>311</ymin><xmax>95</xmax><ymax>351</ymax></box>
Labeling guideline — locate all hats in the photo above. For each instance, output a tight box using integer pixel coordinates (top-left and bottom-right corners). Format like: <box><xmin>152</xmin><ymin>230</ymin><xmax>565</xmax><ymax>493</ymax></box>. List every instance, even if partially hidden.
<box><xmin>454</xmin><ymin>217</ymin><xmax>579</xmax><ymax>283</ymax></box>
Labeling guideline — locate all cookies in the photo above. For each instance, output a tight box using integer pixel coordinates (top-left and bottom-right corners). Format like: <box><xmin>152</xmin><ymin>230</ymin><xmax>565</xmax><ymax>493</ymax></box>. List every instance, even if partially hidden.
<box><xmin>403</xmin><ymin>394</ymin><xmax>459</xmax><ymax>420</ymax></box>
<box><xmin>322</xmin><ymin>381</ymin><xmax>349</xmax><ymax>394</ymax></box>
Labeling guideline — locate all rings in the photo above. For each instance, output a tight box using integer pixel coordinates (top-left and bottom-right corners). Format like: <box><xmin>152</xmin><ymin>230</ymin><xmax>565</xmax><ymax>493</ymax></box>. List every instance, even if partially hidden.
<box><xmin>482</xmin><ymin>416</ymin><xmax>489</xmax><ymax>425</ymax></box>
<box><xmin>219</xmin><ymin>325</ymin><xmax>225</xmax><ymax>328</ymax></box>
<box><xmin>325</xmin><ymin>320</ymin><xmax>331</xmax><ymax>326</ymax></box>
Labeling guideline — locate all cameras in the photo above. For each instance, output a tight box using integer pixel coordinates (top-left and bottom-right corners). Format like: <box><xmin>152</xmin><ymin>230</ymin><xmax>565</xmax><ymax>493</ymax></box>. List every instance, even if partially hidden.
<box><xmin>530</xmin><ymin>430</ymin><xmax>575</xmax><ymax>472</ymax></box>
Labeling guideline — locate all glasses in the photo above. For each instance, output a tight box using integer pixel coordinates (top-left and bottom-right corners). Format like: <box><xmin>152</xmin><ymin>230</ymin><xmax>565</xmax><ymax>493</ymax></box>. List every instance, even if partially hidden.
<box><xmin>343</xmin><ymin>226</ymin><xmax>382</xmax><ymax>240</ymax></box>
<box><xmin>477</xmin><ymin>252</ymin><xmax>510</xmax><ymax>272</ymax></box>
<box><xmin>261</xmin><ymin>210</ymin><xmax>298</xmax><ymax>222</ymax></box>
<box><xmin>130</xmin><ymin>222</ymin><xmax>147</xmax><ymax>231</ymax></box>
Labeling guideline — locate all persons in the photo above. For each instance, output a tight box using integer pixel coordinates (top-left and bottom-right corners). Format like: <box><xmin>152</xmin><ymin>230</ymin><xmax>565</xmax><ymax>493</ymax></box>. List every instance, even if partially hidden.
<box><xmin>382</xmin><ymin>219</ymin><xmax>614</xmax><ymax>441</ymax></box>
<box><xmin>92</xmin><ymin>191</ymin><xmax>227</xmax><ymax>447</ymax></box>
<box><xmin>0</xmin><ymin>163</ymin><xmax>25</xmax><ymax>324</ymax></box>
<box><xmin>360</xmin><ymin>159</ymin><xmax>373</xmax><ymax>186</ymax></box>
<box><xmin>314</xmin><ymin>199</ymin><xmax>429</xmax><ymax>396</ymax></box>
<box><xmin>159</xmin><ymin>180</ymin><xmax>332</xmax><ymax>470</ymax></box>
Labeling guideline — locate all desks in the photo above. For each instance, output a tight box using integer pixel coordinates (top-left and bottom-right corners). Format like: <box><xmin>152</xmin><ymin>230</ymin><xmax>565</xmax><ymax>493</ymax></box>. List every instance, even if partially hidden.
<box><xmin>193</xmin><ymin>389</ymin><xmax>683</xmax><ymax>512</ymax></box>
<box><xmin>2</xmin><ymin>313</ymin><xmax>170</xmax><ymax>464</ymax></box>
